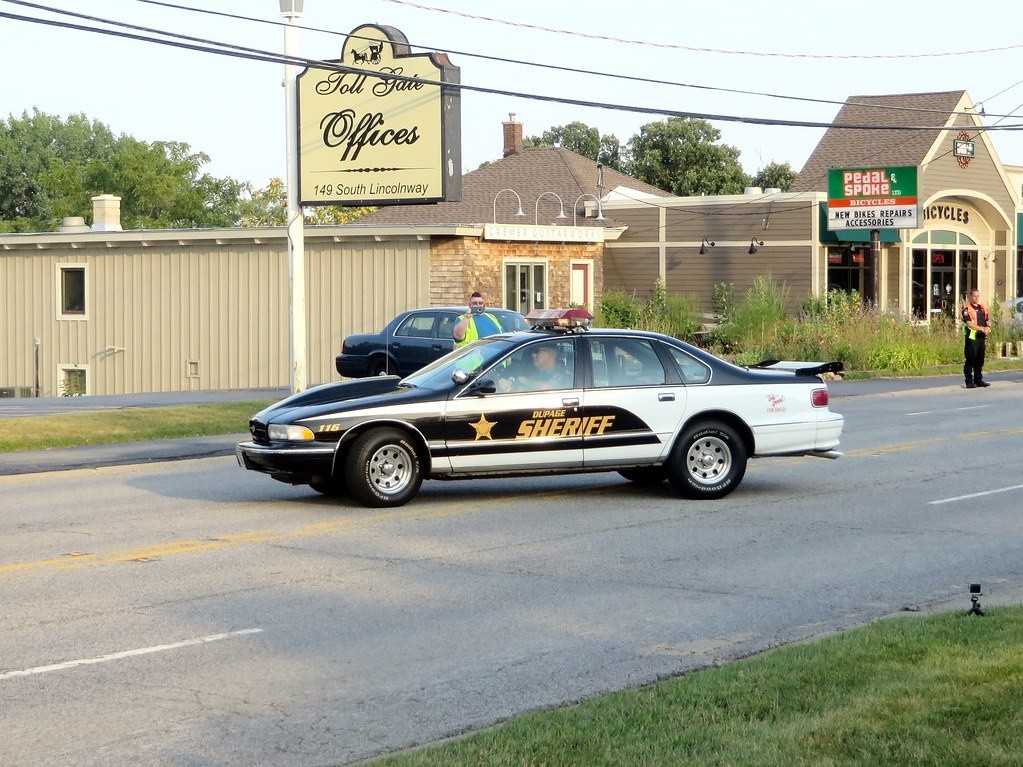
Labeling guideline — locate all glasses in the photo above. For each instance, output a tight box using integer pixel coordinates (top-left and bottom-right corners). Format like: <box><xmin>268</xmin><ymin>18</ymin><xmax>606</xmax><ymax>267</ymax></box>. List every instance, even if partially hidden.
<box><xmin>532</xmin><ymin>349</ymin><xmax>545</xmax><ymax>354</ymax></box>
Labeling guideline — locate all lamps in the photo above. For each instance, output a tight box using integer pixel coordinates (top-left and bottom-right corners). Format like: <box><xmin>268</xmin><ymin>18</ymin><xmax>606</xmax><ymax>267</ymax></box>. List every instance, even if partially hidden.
<box><xmin>748</xmin><ymin>237</ymin><xmax>764</xmax><ymax>254</ymax></box>
<box><xmin>964</xmin><ymin>101</ymin><xmax>985</xmax><ymax>117</ymax></box>
<box><xmin>699</xmin><ymin>237</ymin><xmax>715</xmax><ymax>254</ymax></box>
<box><xmin>534</xmin><ymin>192</ymin><xmax>568</xmax><ymax>225</ymax></box>
<box><xmin>493</xmin><ymin>189</ymin><xmax>528</xmax><ymax>224</ymax></box>
<box><xmin>984</xmin><ymin>250</ymin><xmax>999</xmax><ymax>264</ymax></box>
<box><xmin>573</xmin><ymin>193</ymin><xmax>608</xmax><ymax>226</ymax></box>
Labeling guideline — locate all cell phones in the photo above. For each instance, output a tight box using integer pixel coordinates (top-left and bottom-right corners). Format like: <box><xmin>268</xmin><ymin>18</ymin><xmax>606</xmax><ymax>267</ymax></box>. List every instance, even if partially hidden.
<box><xmin>471</xmin><ymin>307</ymin><xmax>483</xmax><ymax>314</ymax></box>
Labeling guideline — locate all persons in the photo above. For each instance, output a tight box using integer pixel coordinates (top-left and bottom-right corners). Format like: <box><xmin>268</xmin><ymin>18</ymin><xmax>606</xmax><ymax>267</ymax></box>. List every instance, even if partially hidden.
<box><xmin>962</xmin><ymin>289</ymin><xmax>990</xmax><ymax>388</ymax></box>
<box><xmin>498</xmin><ymin>343</ymin><xmax>568</xmax><ymax>392</ymax></box>
<box><xmin>452</xmin><ymin>292</ymin><xmax>511</xmax><ymax>375</ymax></box>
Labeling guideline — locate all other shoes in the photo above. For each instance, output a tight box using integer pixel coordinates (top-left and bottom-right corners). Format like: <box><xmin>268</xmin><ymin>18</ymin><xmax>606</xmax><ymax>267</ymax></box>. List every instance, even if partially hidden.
<box><xmin>966</xmin><ymin>382</ymin><xmax>977</xmax><ymax>388</ymax></box>
<box><xmin>974</xmin><ymin>381</ymin><xmax>990</xmax><ymax>387</ymax></box>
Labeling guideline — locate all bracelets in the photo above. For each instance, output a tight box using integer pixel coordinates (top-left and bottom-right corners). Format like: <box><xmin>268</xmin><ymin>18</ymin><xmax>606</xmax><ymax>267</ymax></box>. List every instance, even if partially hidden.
<box><xmin>508</xmin><ymin>376</ymin><xmax>515</xmax><ymax>382</ymax></box>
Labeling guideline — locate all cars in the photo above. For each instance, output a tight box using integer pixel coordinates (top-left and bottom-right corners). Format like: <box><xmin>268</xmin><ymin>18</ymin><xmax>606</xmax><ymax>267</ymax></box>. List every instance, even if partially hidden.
<box><xmin>333</xmin><ymin>304</ymin><xmax>572</xmax><ymax>382</ymax></box>
<box><xmin>235</xmin><ymin>307</ymin><xmax>847</xmax><ymax>511</ymax></box>
<box><xmin>987</xmin><ymin>297</ymin><xmax>1023</xmax><ymax>324</ymax></box>
